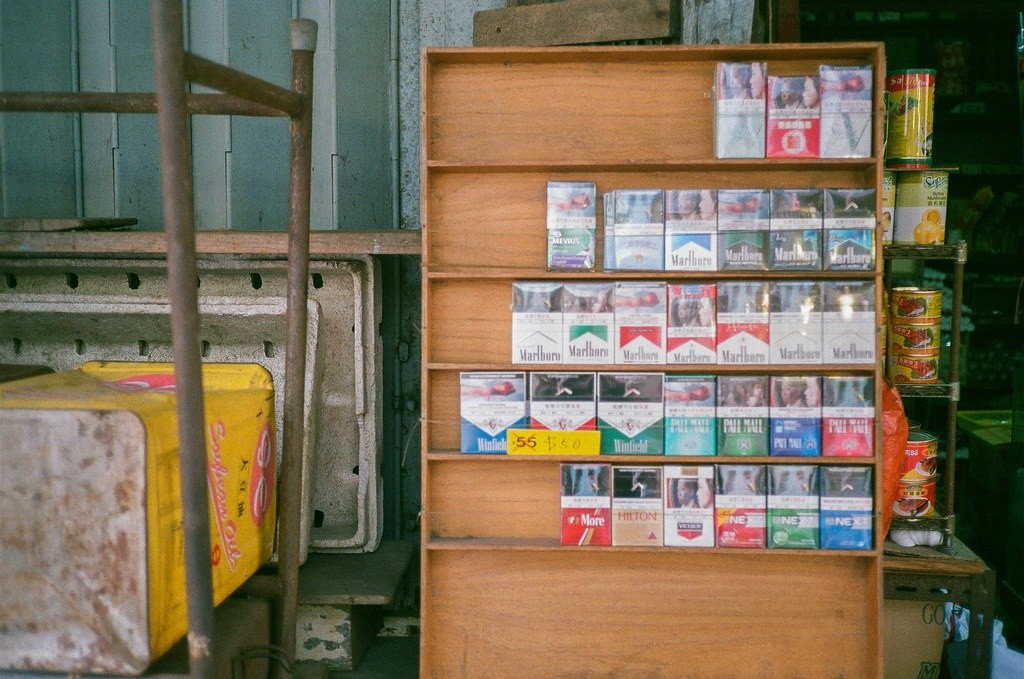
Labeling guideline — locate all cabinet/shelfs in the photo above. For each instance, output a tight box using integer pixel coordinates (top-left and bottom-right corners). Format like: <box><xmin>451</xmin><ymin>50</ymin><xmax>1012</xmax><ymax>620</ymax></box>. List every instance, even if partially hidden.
<box><xmin>881</xmin><ymin>240</ymin><xmax>971</xmax><ymax>559</ymax></box>
<box><xmin>417</xmin><ymin>41</ymin><xmax>886</xmax><ymax>679</ymax></box>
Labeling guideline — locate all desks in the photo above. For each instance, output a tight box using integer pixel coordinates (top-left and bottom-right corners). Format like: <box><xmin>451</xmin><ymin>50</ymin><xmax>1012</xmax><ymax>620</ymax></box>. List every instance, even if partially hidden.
<box><xmin>883</xmin><ymin>538</ymin><xmax>998</xmax><ymax>679</ymax></box>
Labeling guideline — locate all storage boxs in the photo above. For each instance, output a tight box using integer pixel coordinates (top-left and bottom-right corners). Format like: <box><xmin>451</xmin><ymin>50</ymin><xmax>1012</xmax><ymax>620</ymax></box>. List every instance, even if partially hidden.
<box><xmin>884</xmin><ymin>589</ymin><xmax>946</xmax><ymax>679</ymax></box>
